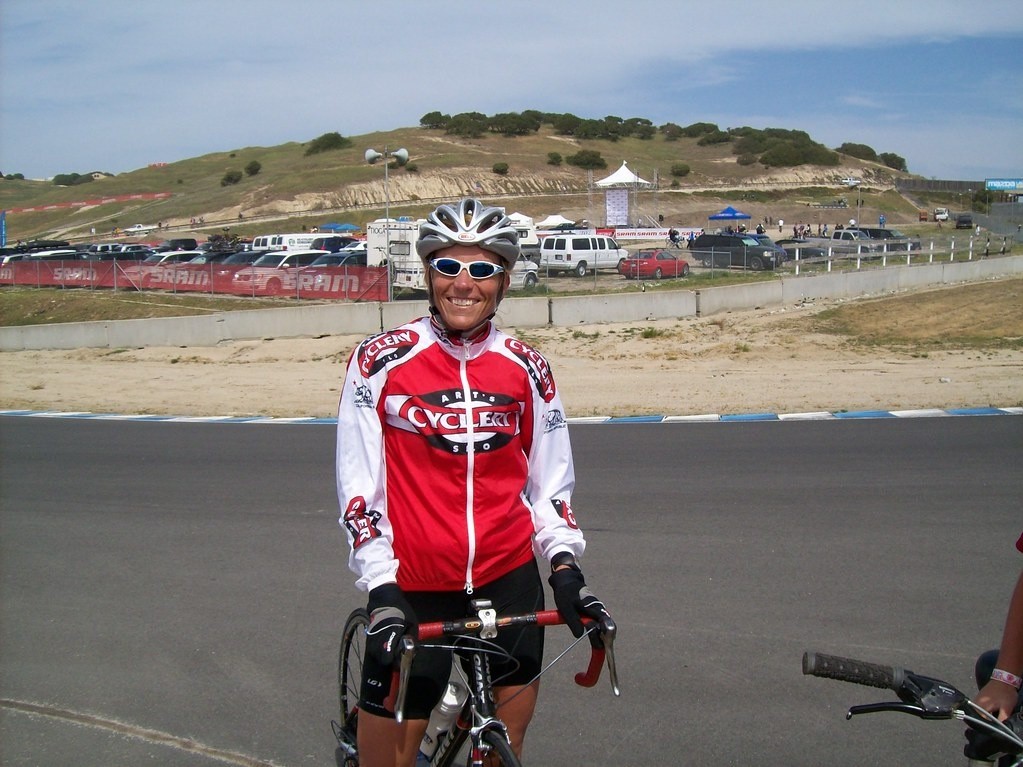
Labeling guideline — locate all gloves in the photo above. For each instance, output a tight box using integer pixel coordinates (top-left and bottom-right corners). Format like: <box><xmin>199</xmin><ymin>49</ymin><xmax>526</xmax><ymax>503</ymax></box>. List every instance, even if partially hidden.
<box><xmin>365</xmin><ymin>582</ymin><xmax>420</xmax><ymax>673</ymax></box>
<box><xmin>547</xmin><ymin>568</ymin><xmax>617</xmax><ymax>650</ymax></box>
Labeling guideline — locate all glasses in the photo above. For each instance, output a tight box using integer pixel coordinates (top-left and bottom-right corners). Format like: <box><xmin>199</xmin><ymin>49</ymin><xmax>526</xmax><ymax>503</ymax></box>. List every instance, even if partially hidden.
<box><xmin>429</xmin><ymin>257</ymin><xmax>505</xmax><ymax>279</ymax></box>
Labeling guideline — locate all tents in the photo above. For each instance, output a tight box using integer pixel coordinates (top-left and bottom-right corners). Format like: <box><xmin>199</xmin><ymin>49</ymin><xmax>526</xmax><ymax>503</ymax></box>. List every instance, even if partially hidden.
<box><xmin>595</xmin><ymin>160</ymin><xmax>651</xmax><ymax>191</ymax></box>
<box><xmin>707</xmin><ymin>206</ymin><xmax>751</xmax><ymax>233</ymax></box>
<box><xmin>508</xmin><ymin>212</ymin><xmax>533</xmax><ymax>226</ymax></box>
<box><xmin>534</xmin><ymin>215</ymin><xmax>575</xmax><ymax>226</ymax></box>
<box><xmin>318</xmin><ymin>222</ymin><xmax>362</xmax><ymax>233</ymax></box>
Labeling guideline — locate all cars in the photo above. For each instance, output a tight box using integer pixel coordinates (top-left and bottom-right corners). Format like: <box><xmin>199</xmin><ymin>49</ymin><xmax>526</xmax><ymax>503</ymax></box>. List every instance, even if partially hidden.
<box><xmin>718</xmin><ymin>232</ymin><xmax>788</xmax><ymax>264</ymax></box>
<box><xmin>774</xmin><ymin>239</ymin><xmax>832</xmax><ymax>265</ymax></box>
<box><xmin>619</xmin><ymin>247</ymin><xmax>689</xmax><ymax>281</ymax></box>
<box><xmin>0</xmin><ymin>232</ymin><xmax>368</xmax><ymax>298</ymax></box>
<box><xmin>955</xmin><ymin>216</ymin><xmax>973</xmax><ymax>229</ymax></box>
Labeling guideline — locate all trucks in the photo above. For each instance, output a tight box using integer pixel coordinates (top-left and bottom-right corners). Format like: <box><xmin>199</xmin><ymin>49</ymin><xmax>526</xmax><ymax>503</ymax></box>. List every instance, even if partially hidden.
<box><xmin>366</xmin><ymin>219</ymin><xmax>540</xmax><ymax>293</ymax></box>
<box><xmin>918</xmin><ymin>207</ymin><xmax>950</xmax><ymax>223</ymax></box>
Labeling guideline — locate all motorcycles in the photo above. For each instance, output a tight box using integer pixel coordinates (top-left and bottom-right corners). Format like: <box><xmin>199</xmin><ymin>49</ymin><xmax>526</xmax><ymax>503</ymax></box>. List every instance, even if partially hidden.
<box><xmin>666</xmin><ymin>237</ymin><xmax>684</xmax><ymax>250</ymax></box>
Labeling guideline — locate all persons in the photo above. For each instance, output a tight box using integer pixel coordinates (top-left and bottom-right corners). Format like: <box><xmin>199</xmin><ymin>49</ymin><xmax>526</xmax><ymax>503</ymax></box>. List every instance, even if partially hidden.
<box><xmin>818</xmin><ymin>223</ymin><xmax>827</xmax><ymax>237</ymax></box>
<box><xmin>779</xmin><ymin>218</ymin><xmax>783</xmax><ymax>231</ymax></box>
<box><xmin>976</xmin><ymin>224</ymin><xmax>980</xmax><ymax>241</ymax></box>
<box><xmin>938</xmin><ymin>218</ymin><xmax>941</xmax><ymax>227</ymax></box>
<box><xmin>158</xmin><ymin>221</ymin><xmax>169</xmax><ymax>228</ymax></box>
<box><xmin>764</xmin><ymin>216</ymin><xmax>772</xmax><ymax>225</ymax></box>
<box><xmin>974</xmin><ymin>532</ymin><xmax>1023</xmax><ymax>724</ymax></box>
<box><xmin>191</xmin><ymin>217</ymin><xmax>204</xmax><ymax>224</ymax></box>
<box><xmin>687</xmin><ymin>231</ymin><xmax>694</xmax><ymax>249</ymax></box>
<box><xmin>756</xmin><ymin>223</ymin><xmax>765</xmax><ymax>234</ymax></box>
<box><xmin>239</xmin><ymin>214</ymin><xmax>242</xmax><ymax>219</ymax></box>
<box><xmin>849</xmin><ymin>217</ymin><xmax>856</xmax><ymax>227</ymax></box>
<box><xmin>879</xmin><ymin>214</ymin><xmax>886</xmax><ymax>228</ymax></box>
<box><xmin>835</xmin><ymin>223</ymin><xmax>844</xmax><ymax>230</ymax></box>
<box><xmin>336</xmin><ymin>200</ymin><xmax>611</xmax><ymax>767</ymax></box>
<box><xmin>793</xmin><ymin>221</ymin><xmax>811</xmax><ymax>239</ymax></box>
<box><xmin>725</xmin><ymin>223</ymin><xmax>746</xmax><ymax>234</ymax></box>
<box><xmin>16</xmin><ymin>237</ymin><xmax>38</xmax><ymax>245</ymax></box>
<box><xmin>701</xmin><ymin>228</ymin><xmax>705</xmax><ymax>234</ymax></box>
<box><xmin>669</xmin><ymin>226</ymin><xmax>682</xmax><ymax>249</ymax></box>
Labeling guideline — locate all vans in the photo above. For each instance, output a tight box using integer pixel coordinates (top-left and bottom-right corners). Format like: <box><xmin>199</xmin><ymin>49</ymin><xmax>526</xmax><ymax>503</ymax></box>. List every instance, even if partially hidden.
<box><xmin>846</xmin><ymin>226</ymin><xmax>921</xmax><ymax>258</ymax></box>
<box><xmin>270</xmin><ymin>234</ymin><xmax>347</xmax><ymax>252</ymax></box>
<box><xmin>539</xmin><ymin>235</ymin><xmax>629</xmax><ymax>278</ymax></box>
<box><xmin>310</xmin><ymin>237</ymin><xmax>359</xmax><ymax>253</ymax></box>
<box><xmin>251</xmin><ymin>236</ymin><xmax>272</xmax><ymax>254</ymax></box>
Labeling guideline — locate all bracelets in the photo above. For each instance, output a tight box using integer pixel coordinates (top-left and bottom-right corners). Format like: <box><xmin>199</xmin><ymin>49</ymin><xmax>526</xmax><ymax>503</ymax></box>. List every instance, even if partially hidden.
<box><xmin>990</xmin><ymin>668</ymin><xmax>1022</xmax><ymax>689</ymax></box>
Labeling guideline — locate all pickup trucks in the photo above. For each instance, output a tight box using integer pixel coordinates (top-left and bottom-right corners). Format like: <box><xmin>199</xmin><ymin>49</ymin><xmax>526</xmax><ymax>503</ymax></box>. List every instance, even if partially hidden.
<box><xmin>805</xmin><ymin>230</ymin><xmax>883</xmax><ymax>261</ymax></box>
<box><xmin>123</xmin><ymin>224</ymin><xmax>153</xmax><ymax>236</ymax></box>
<box><xmin>842</xmin><ymin>178</ymin><xmax>861</xmax><ymax>183</ymax></box>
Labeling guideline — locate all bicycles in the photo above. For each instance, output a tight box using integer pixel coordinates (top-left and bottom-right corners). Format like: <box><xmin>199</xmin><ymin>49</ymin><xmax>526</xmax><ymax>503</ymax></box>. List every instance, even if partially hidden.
<box><xmin>800</xmin><ymin>647</ymin><xmax>1023</xmax><ymax>767</ymax></box>
<box><xmin>331</xmin><ymin>608</ymin><xmax>622</xmax><ymax>767</ymax></box>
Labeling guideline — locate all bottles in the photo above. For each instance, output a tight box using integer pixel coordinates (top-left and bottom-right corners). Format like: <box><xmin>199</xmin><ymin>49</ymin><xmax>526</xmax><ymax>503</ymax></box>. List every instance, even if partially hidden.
<box><xmin>419</xmin><ymin>681</ymin><xmax>467</xmax><ymax>755</ymax></box>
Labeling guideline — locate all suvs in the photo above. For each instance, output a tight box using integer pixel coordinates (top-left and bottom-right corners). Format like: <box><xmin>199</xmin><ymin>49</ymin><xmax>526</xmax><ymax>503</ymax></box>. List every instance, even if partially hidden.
<box><xmin>690</xmin><ymin>233</ymin><xmax>782</xmax><ymax>272</ymax></box>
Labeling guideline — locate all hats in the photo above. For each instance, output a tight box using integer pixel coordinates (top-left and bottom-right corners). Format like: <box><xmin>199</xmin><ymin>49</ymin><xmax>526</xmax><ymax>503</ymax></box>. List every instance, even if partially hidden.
<box><xmin>415</xmin><ymin>199</ymin><xmax>521</xmax><ymax>273</ymax></box>
